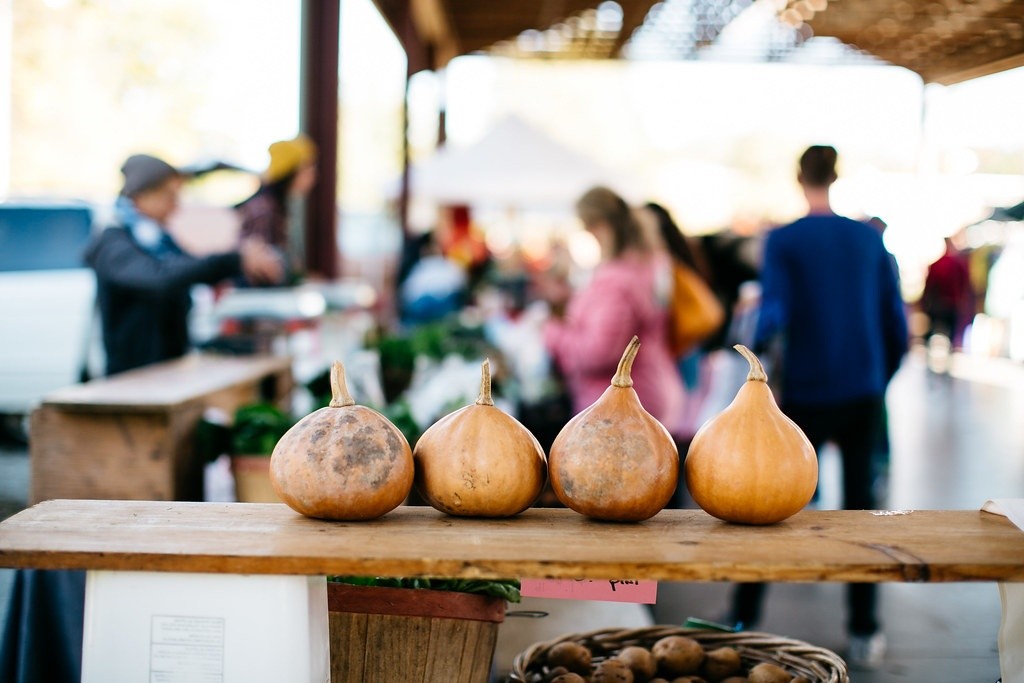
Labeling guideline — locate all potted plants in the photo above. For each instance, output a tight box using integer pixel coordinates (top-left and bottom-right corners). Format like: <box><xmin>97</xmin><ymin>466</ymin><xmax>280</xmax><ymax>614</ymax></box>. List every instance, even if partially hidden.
<box><xmin>331</xmin><ymin>576</ymin><xmax>521</xmax><ymax>683</ymax></box>
<box><xmin>228</xmin><ymin>400</ymin><xmax>296</xmax><ymax>506</ymax></box>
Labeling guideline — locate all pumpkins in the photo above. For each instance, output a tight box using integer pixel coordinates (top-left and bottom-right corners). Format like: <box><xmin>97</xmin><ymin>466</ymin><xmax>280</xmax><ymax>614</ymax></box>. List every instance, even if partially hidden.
<box><xmin>415</xmin><ymin>360</ymin><xmax>548</xmax><ymax>519</ymax></box>
<box><xmin>686</xmin><ymin>345</ymin><xmax>819</xmax><ymax>521</ymax></box>
<box><xmin>270</xmin><ymin>359</ymin><xmax>414</xmax><ymax>517</ymax></box>
<box><xmin>550</xmin><ymin>335</ymin><xmax>681</xmax><ymax>520</ymax></box>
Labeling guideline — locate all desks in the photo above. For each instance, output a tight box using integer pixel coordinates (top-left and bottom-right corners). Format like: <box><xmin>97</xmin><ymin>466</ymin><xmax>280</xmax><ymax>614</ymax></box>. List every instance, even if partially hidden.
<box><xmin>0</xmin><ymin>497</ymin><xmax>1024</xmax><ymax>683</ymax></box>
<box><xmin>32</xmin><ymin>350</ymin><xmax>297</xmax><ymax>499</ymax></box>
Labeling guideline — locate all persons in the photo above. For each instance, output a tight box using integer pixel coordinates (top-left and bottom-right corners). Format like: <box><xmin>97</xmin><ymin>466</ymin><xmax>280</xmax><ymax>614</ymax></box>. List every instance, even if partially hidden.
<box><xmin>82</xmin><ymin>153</ymin><xmax>286</xmax><ymax>388</ymax></box>
<box><xmin>919</xmin><ymin>237</ymin><xmax>975</xmax><ymax>354</ymax></box>
<box><xmin>534</xmin><ymin>186</ymin><xmax>761</xmax><ymax>510</ymax></box>
<box><xmin>225</xmin><ymin>137</ymin><xmax>313</xmax><ymax>404</ymax></box>
<box><xmin>716</xmin><ymin>144</ymin><xmax>909</xmax><ymax>664</ymax></box>
<box><xmin>377</xmin><ymin>205</ymin><xmax>497</xmax><ymax>405</ymax></box>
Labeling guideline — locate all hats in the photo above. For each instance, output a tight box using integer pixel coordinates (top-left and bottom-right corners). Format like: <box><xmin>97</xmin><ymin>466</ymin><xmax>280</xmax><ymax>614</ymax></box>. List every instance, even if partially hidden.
<box><xmin>267</xmin><ymin>136</ymin><xmax>313</xmax><ymax>178</ymax></box>
<box><xmin>120</xmin><ymin>155</ymin><xmax>180</xmax><ymax>196</ymax></box>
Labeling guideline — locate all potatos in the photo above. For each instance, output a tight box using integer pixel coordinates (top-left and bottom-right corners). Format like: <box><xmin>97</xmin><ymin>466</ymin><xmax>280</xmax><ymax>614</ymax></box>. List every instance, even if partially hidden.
<box><xmin>546</xmin><ymin>638</ymin><xmax>810</xmax><ymax>683</ymax></box>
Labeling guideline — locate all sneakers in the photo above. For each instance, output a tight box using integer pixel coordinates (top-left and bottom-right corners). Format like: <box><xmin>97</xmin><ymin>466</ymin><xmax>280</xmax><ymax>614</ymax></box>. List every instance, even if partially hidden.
<box><xmin>850</xmin><ymin>629</ymin><xmax>891</xmax><ymax>671</ymax></box>
